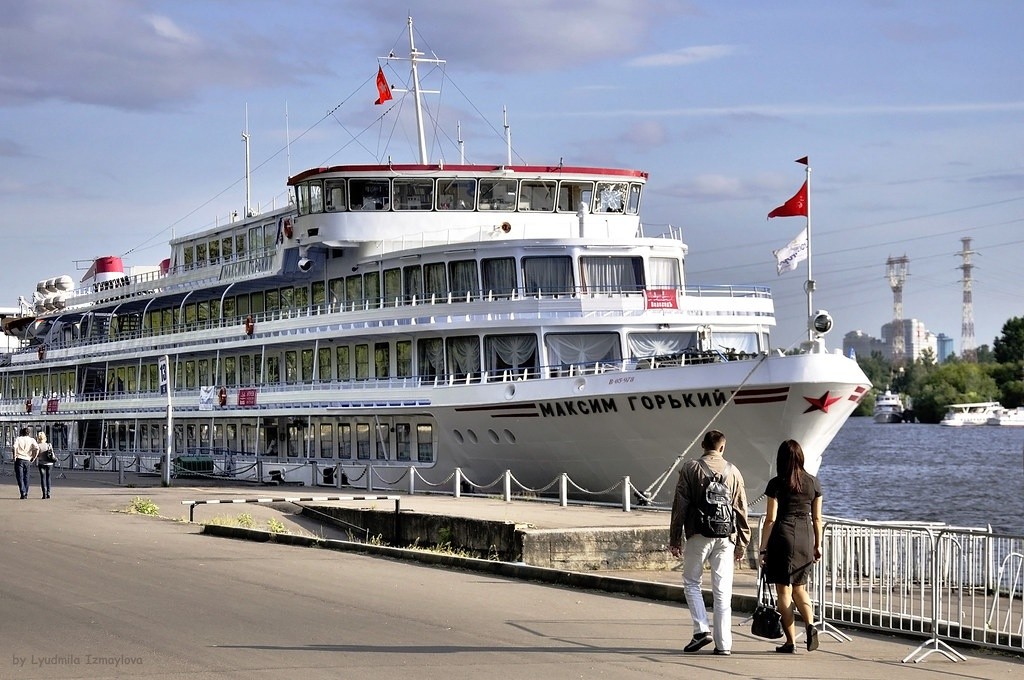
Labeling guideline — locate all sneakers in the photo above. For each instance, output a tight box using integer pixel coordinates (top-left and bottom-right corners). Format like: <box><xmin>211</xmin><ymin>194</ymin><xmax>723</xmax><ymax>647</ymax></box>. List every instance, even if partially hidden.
<box><xmin>714</xmin><ymin>647</ymin><xmax>730</xmax><ymax>655</ymax></box>
<box><xmin>684</xmin><ymin>632</ymin><xmax>713</xmax><ymax>652</ymax></box>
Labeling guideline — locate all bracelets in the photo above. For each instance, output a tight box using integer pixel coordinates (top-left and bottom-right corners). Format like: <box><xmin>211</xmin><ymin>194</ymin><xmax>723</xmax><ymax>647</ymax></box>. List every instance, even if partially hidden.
<box><xmin>760</xmin><ymin>551</ymin><xmax>766</xmax><ymax>555</ymax></box>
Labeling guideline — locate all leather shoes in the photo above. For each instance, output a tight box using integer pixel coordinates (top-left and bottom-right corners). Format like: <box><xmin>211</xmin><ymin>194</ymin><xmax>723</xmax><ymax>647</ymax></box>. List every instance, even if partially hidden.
<box><xmin>806</xmin><ymin>624</ymin><xmax>819</xmax><ymax>651</ymax></box>
<box><xmin>776</xmin><ymin>642</ymin><xmax>794</xmax><ymax>653</ymax></box>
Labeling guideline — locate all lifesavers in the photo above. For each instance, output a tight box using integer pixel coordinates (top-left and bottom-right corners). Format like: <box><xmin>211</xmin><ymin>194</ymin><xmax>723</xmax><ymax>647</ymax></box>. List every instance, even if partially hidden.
<box><xmin>246</xmin><ymin>316</ymin><xmax>253</xmax><ymax>335</ymax></box>
<box><xmin>219</xmin><ymin>387</ymin><xmax>226</xmax><ymax>406</ymax></box>
<box><xmin>38</xmin><ymin>344</ymin><xmax>44</xmax><ymax>359</ymax></box>
<box><xmin>284</xmin><ymin>221</ymin><xmax>293</xmax><ymax>238</ymax></box>
<box><xmin>26</xmin><ymin>400</ymin><xmax>31</xmax><ymax>411</ymax></box>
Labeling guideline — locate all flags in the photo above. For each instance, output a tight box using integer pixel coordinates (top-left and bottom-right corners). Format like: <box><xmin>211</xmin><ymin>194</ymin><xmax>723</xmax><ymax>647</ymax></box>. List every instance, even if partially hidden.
<box><xmin>375</xmin><ymin>64</ymin><xmax>394</xmax><ymax>105</ymax></box>
<box><xmin>774</xmin><ymin>226</ymin><xmax>808</xmax><ymax>276</ymax></box>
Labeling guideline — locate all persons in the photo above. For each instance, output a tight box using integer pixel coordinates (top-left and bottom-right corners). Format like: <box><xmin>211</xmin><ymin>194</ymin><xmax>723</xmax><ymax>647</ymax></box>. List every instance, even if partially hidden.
<box><xmin>111</xmin><ymin>373</ymin><xmax>123</xmax><ymax>391</ymax></box>
<box><xmin>670</xmin><ymin>429</ymin><xmax>751</xmax><ymax>655</ymax></box>
<box><xmin>36</xmin><ymin>432</ymin><xmax>53</xmax><ymax>499</ymax></box>
<box><xmin>26</xmin><ymin>397</ymin><xmax>31</xmax><ymax>413</ymax></box>
<box><xmin>12</xmin><ymin>428</ymin><xmax>40</xmax><ymax>499</ymax></box>
<box><xmin>758</xmin><ymin>438</ymin><xmax>823</xmax><ymax>653</ymax></box>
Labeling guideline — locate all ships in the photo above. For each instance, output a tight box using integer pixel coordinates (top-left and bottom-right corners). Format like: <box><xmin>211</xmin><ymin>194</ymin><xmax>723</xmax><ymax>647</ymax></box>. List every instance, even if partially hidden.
<box><xmin>1</xmin><ymin>16</ymin><xmax>878</xmax><ymax>518</ymax></box>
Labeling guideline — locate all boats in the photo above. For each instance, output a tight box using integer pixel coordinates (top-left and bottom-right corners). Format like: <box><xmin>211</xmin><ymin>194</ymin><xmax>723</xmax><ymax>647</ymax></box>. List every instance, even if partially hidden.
<box><xmin>938</xmin><ymin>398</ymin><xmax>1006</xmax><ymax>428</ymax></box>
<box><xmin>986</xmin><ymin>406</ymin><xmax>1024</xmax><ymax>428</ymax></box>
<box><xmin>873</xmin><ymin>382</ymin><xmax>907</xmax><ymax>425</ymax></box>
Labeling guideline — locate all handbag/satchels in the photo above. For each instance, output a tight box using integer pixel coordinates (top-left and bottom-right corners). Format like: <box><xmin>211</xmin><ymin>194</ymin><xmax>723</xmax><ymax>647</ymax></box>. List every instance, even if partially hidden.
<box><xmin>751</xmin><ymin>568</ymin><xmax>784</xmax><ymax>639</ymax></box>
<box><xmin>43</xmin><ymin>443</ymin><xmax>56</xmax><ymax>463</ymax></box>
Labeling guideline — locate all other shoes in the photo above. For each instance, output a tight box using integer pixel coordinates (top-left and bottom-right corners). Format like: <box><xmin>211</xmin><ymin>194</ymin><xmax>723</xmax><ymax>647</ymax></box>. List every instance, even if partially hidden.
<box><xmin>42</xmin><ymin>497</ymin><xmax>46</xmax><ymax>499</ymax></box>
<box><xmin>25</xmin><ymin>494</ymin><xmax>28</xmax><ymax>499</ymax></box>
<box><xmin>47</xmin><ymin>495</ymin><xmax>51</xmax><ymax>498</ymax></box>
<box><xmin>20</xmin><ymin>493</ymin><xmax>25</xmax><ymax>499</ymax></box>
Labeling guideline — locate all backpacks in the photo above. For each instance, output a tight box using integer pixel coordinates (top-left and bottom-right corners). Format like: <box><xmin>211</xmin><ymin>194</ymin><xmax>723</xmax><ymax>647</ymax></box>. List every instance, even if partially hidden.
<box><xmin>694</xmin><ymin>458</ymin><xmax>736</xmax><ymax>546</ymax></box>
